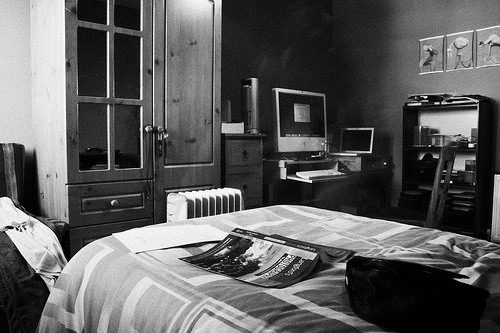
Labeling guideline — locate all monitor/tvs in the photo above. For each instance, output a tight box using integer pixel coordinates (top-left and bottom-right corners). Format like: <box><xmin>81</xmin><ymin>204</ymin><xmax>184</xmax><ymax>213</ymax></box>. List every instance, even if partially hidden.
<box><xmin>271</xmin><ymin>87</ymin><xmax>328</xmax><ymax>160</ymax></box>
<box><xmin>340</xmin><ymin>127</ymin><xmax>374</xmax><ymax>154</ymax></box>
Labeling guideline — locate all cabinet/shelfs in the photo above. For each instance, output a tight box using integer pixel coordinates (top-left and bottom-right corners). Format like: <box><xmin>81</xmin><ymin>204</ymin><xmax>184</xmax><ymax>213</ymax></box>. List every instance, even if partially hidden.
<box><xmin>401</xmin><ymin>100</ymin><xmax>495</xmax><ymax>240</ymax></box>
<box><xmin>65</xmin><ymin>0</ymin><xmax>222</xmax><ymax>260</ymax></box>
<box><xmin>221</xmin><ymin>134</ymin><xmax>267</xmax><ymax>210</ymax></box>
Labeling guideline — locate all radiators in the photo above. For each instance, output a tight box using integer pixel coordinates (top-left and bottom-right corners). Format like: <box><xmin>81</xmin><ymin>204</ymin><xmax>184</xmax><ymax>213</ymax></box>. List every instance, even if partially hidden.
<box><xmin>167</xmin><ymin>187</ymin><xmax>243</xmax><ymax>222</ymax></box>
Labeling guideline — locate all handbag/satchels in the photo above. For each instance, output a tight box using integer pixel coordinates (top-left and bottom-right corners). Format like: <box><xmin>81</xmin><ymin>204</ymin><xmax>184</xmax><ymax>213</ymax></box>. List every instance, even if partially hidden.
<box><xmin>344</xmin><ymin>255</ymin><xmax>492</xmax><ymax>333</ymax></box>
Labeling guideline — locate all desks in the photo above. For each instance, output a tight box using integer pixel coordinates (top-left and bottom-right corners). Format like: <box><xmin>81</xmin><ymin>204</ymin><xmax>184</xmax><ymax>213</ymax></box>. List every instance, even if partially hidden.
<box><xmin>263</xmin><ymin>159</ymin><xmax>394</xmax><ymax>208</ymax></box>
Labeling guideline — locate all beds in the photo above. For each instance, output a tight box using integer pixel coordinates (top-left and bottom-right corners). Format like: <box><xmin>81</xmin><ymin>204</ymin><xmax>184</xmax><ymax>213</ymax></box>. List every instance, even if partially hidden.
<box><xmin>35</xmin><ymin>205</ymin><xmax>500</xmax><ymax>333</ymax></box>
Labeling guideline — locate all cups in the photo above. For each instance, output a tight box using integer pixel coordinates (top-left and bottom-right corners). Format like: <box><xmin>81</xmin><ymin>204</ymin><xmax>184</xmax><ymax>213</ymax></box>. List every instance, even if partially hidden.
<box><xmin>321</xmin><ymin>140</ymin><xmax>329</xmax><ymax>158</ymax></box>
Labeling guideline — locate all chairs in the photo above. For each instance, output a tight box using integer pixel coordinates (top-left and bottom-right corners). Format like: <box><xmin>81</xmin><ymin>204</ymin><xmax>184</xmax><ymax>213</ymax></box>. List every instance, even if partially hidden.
<box><xmin>0</xmin><ymin>142</ymin><xmax>69</xmax><ymax>333</ymax></box>
<box><xmin>372</xmin><ymin>146</ymin><xmax>456</xmax><ymax>231</ymax></box>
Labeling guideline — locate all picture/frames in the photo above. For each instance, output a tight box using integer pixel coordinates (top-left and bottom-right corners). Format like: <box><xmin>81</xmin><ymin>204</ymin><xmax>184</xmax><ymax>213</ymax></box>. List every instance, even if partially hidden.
<box><xmin>474</xmin><ymin>25</ymin><xmax>500</xmax><ymax>69</ymax></box>
<box><xmin>419</xmin><ymin>35</ymin><xmax>444</xmax><ymax>75</ymax></box>
<box><xmin>445</xmin><ymin>30</ymin><xmax>474</xmax><ymax>73</ymax></box>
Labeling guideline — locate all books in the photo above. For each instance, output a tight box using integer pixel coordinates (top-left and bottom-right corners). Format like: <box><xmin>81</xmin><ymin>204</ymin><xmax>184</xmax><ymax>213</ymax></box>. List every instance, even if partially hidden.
<box><xmin>179</xmin><ymin>228</ymin><xmax>353</xmax><ymax>289</ymax></box>
<box><xmin>452</xmin><ymin>199</ymin><xmax>474</xmax><ymax>210</ymax></box>
<box><xmin>413</xmin><ymin>125</ymin><xmax>478</xmax><ymax>147</ymax></box>
<box><xmin>440</xmin><ymin>160</ymin><xmax>476</xmax><ymax>184</ymax></box>
<box><xmin>296</xmin><ymin>169</ymin><xmax>341</xmax><ymax>179</ymax></box>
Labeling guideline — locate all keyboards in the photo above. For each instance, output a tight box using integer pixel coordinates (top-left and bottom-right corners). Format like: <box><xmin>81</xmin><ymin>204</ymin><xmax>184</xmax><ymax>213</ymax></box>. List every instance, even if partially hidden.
<box><xmin>296</xmin><ymin>169</ymin><xmax>347</xmax><ymax>180</ymax></box>
